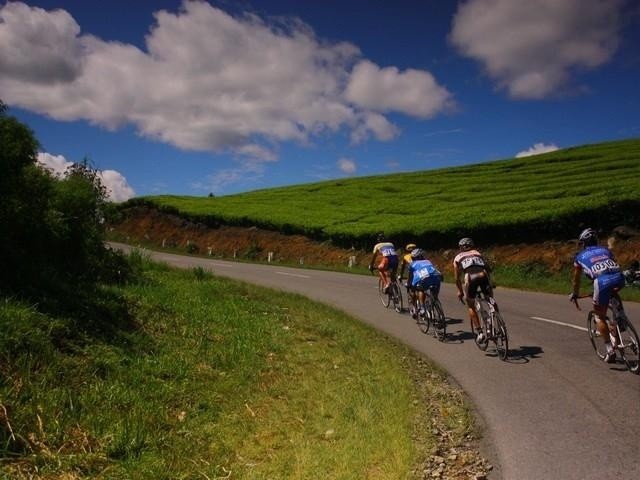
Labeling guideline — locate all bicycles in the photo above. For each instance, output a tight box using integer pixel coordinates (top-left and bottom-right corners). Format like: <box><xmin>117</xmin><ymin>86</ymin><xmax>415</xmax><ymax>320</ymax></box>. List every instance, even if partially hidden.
<box><xmin>371</xmin><ymin>267</ymin><xmax>403</xmax><ymax>313</ymax></box>
<box><xmin>401</xmin><ymin>276</ymin><xmax>446</xmax><ymax>342</ymax></box>
<box><xmin>461</xmin><ymin>286</ymin><xmax>508</xmax><ymax>360</ymax></box>
<box><xmin>574</xmin><ymin>290</ymin><xmax>640</xmax><ymax>373</ymax></box>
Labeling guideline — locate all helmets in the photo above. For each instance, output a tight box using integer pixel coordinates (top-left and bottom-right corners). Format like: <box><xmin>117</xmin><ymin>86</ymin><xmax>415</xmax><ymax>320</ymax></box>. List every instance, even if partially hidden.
<box><xmin>579</xmin><ymin>227</ymin><xmax>597</xmax><ymax>247</ymax></box>
<box><xmin>405</xmin><ymin>244</ymin><xmax>423</xmax><ymax>260</ymax></box>
<box><xmin>376</xmin><ymin>233</ymin><xmax>385</xmax><ymax>242</ymax></box>
<box><xmin>459</xmin><ymin>237</ymin><xmax>474</xmax><ymax>251</ymax></box>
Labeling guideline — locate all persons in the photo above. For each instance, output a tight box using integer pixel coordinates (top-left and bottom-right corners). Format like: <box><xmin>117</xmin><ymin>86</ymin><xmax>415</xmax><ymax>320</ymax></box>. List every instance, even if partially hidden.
<box><xmin>569</xmin><ymin>228</ymin><xmax>626</xmax><ymax>363</ymax></box>
<box><xmin>369</xmin><ymin>231</ymin><xmax>399</xmax><ymax>304</ymax></box>
<box><xmin>405</xmin><ymin>248</ymin><xmax>444</xmax><ymax>323</ymax></box>
<box><xmin>454</xmin><ymin>237</ymin><xmax>499</xmax><ymax>345</ymax></box>
<box><xmin>398</xmin><ymin>243</ymin><xmax>418</xmax><ymax>318</ymax></box>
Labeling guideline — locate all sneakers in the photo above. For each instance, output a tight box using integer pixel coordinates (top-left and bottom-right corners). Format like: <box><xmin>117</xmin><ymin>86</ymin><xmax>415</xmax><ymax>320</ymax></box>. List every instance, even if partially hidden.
<box><xmin>604</xmin><ymin>350</ymin><xmax>616</xmax><ymax>363</ymax></box>
<box><xmin>383</xmin><ymin>285</ymin><xmax>390</xmax><ymax>294</ymax></box>
<box><xmin>476</xmin><ymin>333</ymin><xmax>485</xmax><ymax>344</ymax></box>
<box><xmin>420</xmin><ymin>310</ymin><xmax>425</xmax><ymax>315</ymax></box>
